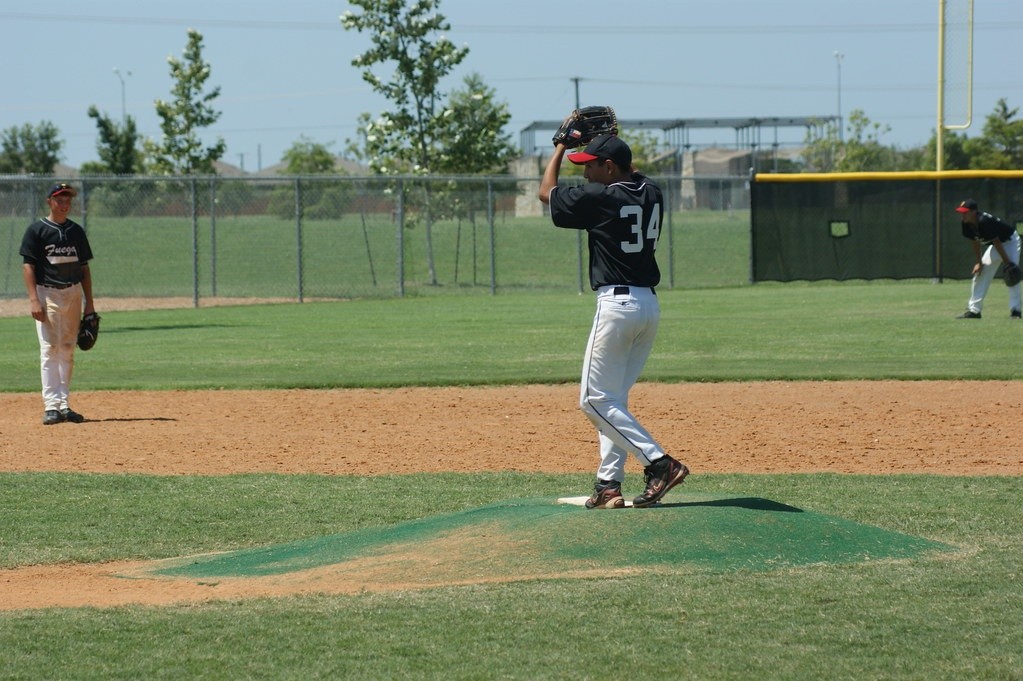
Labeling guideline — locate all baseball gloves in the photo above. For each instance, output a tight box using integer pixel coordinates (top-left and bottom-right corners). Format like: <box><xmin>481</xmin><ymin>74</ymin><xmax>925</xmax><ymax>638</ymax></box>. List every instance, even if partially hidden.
<box><xmin>552</xmin><ymin>106</ymin><xmax>619</xmax><ymax>149</ymax></box>
<box><xmin>1003</xmin><ymin>262</ymin><xmax>1021</xmax><ymax>286</ymax></box>
<box><xmin>77</xmin><ymin>312</ymin><xmax>100</xmax><ymax>351</ymax></box>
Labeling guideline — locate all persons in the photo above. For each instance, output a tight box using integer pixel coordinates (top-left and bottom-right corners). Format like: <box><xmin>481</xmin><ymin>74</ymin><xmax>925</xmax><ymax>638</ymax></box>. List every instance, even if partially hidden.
<box><xmin>955</xmin><ymin>198</ymin><xmax>1022</xmax><ymax>319</ymax></box>
<box><xmin>538</xmin><ymin>105</ymin><xmax>689</xmax><ymax>509</ymax></box>
<box><xmin>19</xmin><ymin>183</ymin><xmax>100</xmax><ymax>425</ymax></box>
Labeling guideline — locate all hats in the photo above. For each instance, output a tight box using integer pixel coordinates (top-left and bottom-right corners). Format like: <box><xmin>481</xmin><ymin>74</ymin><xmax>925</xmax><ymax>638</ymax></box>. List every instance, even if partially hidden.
<box><xmin>48</xmin><ymin>183</ymin><xmax>76</xmax><ymax>198</ymax></box>
<box><xmin>567</xmin><ymin>134</ymin><xmax>632</xmax><ymax>170</ymax></box>
<box><xmin>956</xmin><ymin>199</ymin><xmax>978</xmax><ymax>214</ymax></box>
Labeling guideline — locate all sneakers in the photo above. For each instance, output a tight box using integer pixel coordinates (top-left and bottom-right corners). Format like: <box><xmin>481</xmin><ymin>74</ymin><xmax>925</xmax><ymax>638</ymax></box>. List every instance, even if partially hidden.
<box><xmin>1009</xmin><ymin>310</ymin><xmax>1021</xmax><ymax>319</ymax></box>
<box><xmin>956</xmin><ymin>311</ymin><xmax>980</xmax><ymax>318</ymax></box>
<box><xmin>585</xmin><ymin>479</ymin><xmax>625</xmax><ymax>509</ymax></box>
<box><xmin>633</xmin><ymin>453</ymin><xmax>689</xmax><ymax>508</ymax></box>
<box><xmin>43</xmin><ymin>408</ymin><xmax>83</xmax><ymax>425</ymax></box>
